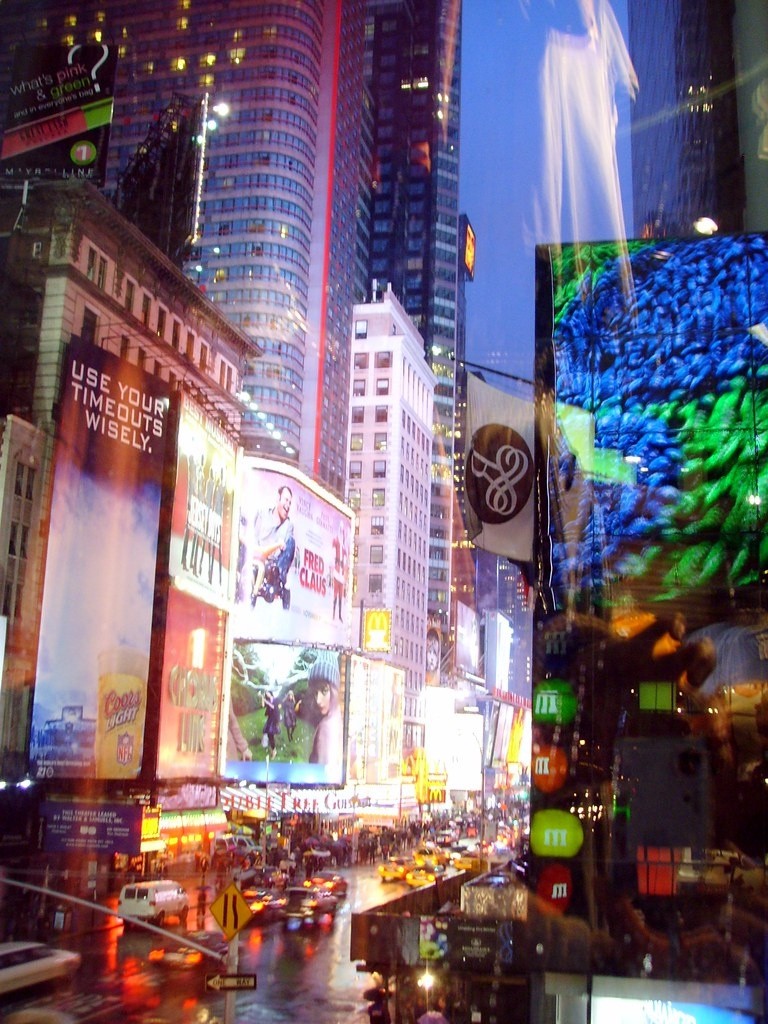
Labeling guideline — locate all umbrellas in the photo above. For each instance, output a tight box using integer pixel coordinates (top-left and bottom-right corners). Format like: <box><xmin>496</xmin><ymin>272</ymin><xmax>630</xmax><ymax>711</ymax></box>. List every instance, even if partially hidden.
<box><xmin>195</xmin><ymin>885</ymin><xmax>211</xmax><ymax>892</ymax></box>
<box><xmin>289</xmin><ymin>832</ymin><xmax>353</xmax><ymax>857</ymax></box>
<box><xmin>418</xmin><ymin>1010</ymin><xmax>448</xmax><ymax>1024</ymax></box>
<box><xmin>363</xmin><ymin>987</ymin><xmax>393</xmax><ymax>1000</ymax></box>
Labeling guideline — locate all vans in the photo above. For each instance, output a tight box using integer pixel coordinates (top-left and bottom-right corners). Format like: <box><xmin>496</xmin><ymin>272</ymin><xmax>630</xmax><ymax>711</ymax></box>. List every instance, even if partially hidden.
<box><xmin>118</xmin><ymin>880</ymin><xmax>189</xmax><ymax>928</ymax></box>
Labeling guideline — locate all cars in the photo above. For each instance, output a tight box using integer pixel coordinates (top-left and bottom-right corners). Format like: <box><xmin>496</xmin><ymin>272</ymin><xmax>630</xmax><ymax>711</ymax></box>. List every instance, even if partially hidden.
<box><xmin>230</xmin><ymin>869</ymin><xmax>350</xmax><ymax>936</ymax></box>
<box><xmin>376</xmin><ymin>825</ymin><xmax>469</xmax><ymax>888</ymax></box>
<box><xmin>0</xmin><ymin>942</ymin><xmax>82</xmax><ymax>995</ymax></box>
<box><xmin>149</xmin><ymin>930</ymin><xmax>246</xmax><ymax>975</ymax></box>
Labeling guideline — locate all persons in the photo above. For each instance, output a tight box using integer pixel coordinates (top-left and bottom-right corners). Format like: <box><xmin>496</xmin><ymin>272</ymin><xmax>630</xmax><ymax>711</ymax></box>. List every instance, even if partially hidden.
<box><xmin>426</xmin><ymin>627</ymin><xmax>439</xmax><ymax>687</ymax></box>
<box><xmin>356</xmin><ymin>798</ymin><xmax>530</xmax><ymax>865</ymax></box>
<box><xmin>368</xmin><ymin>1000</ymin><xmax>391</xmax><ymax>1024</ymax></box>
<box><xmin>194</xmin><ymin>840</ymin><xmax>345</xmax><ymax>918</ymax></box>
<box><xmin>415</xmin><ymin>996</ymin><xmax>449</xmax><ymax>1017</ymax></box>
<box><xmin>226</xmin><ymin>482</ymin><xmax>339</xmax><ymax>770</ymax></box>
